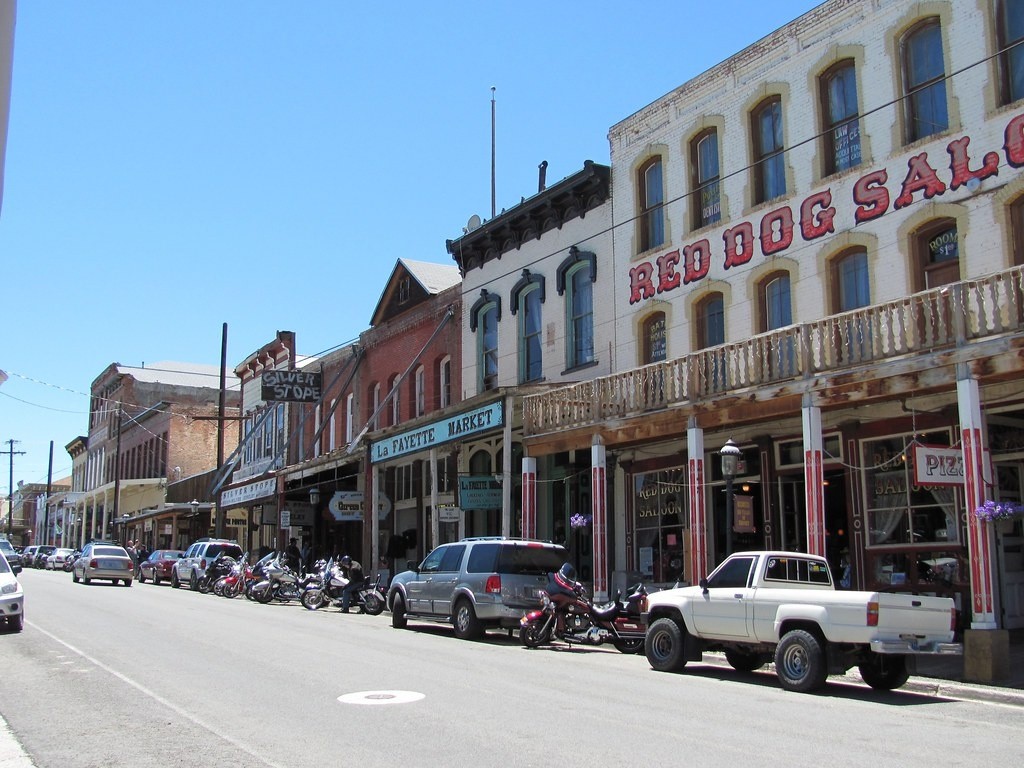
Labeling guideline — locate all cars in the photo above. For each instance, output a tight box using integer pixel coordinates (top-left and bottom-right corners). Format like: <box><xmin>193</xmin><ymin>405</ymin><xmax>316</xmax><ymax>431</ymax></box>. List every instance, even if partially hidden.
<box><xmin>74</xmin><ymin>541</ymin><xmax>115</xmax><ymax>561</ymax></box>
<box><xmin>24</xmin><ymin>546</ymin><xmax>37</xmax><ymax>556</ymax></box>
<box><xmin>138</xmin><ymin>549</ymin><xmax>185</xmax><ymax>585</ymax></box>
<box><xmin>0</xmin><ymin>538</ymin><xmax>23</xmax><ymax>577</ymax></box>
<box><xmin>32</xmin><ymin>545</ymin><xmax>56</xmax><ymax>567</ymax></box>
<box><xmin>0</xmin><ymin>549</ymin><xmax>25</xmax><ymax>631</ymax></box>
<box><xmin>45</xmin><ymin>548</ymin><xmax>74</xmax><ymax>570</ymax></box>
<box><xmin>71</xmin><ymin>545</ymin><xmax>134</xmax><ymax>587</ymax></box>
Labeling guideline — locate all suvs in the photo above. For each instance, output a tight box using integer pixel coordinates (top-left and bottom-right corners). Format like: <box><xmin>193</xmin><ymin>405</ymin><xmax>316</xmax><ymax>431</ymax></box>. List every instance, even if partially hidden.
<box><xmin>385</xmin><ymin>536</ymin><xmax>568</xmax><ymax>640</ymax></box>
<box><xmin>170</xmin><ymin>538</ymin><xmax>245</xmax><ymax>590</ymax></box>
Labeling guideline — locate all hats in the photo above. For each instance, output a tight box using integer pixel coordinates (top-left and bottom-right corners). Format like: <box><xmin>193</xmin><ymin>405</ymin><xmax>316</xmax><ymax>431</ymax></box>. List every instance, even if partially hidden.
<box><xmin>289</xmin><ymin>537</ymin><xmax>299</xmax><ymax>544</ymax></box>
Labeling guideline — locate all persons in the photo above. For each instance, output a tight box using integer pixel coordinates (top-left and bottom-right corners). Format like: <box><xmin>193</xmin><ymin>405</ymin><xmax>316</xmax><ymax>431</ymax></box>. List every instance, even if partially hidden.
<box><xmin>287</xmin><ymin>537</ymin><xmax>303</xmax><ymax>572</ymax></box>
<box><xmin>127</xmin><ymin>538</ymin><xmax>141</xmax><ymax>579</ymax></box>
<box><xmin>139</xmin><ymin>545</ymin><xmax>149</xmax><ymax>569</ymax></box>
<box><xmin>340</xmin><ymin>555</ymin><xmax>364</xmax><ymax>613</ymax></box>
<box><xmin>300</xmin><ymin>541</ymin><xmax>313</xmax><ymax>570</ymax></box>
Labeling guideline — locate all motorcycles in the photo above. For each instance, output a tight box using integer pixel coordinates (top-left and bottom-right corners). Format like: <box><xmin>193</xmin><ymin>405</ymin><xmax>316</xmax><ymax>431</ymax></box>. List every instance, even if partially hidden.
<box><xmin>197</xmin><ymin>551</ymin><xmax>330</xmax><ymax>607</ymax></box>
<box><xmin>300</xmin><ymin>556</ymin><xmax>385</xmax><ymax>616</ymax></box>
<box><xmin>519</xmin><ymin>563</ymin><xmax>650</xmax><ymax>654</ymax></box>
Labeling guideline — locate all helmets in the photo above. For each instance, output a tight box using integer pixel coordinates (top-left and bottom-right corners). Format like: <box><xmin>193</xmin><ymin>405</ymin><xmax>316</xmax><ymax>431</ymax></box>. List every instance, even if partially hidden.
<box><xmin>341</xmin><ymin>555</ymin><xmax>352</xmax><ymax>566</ymax></box>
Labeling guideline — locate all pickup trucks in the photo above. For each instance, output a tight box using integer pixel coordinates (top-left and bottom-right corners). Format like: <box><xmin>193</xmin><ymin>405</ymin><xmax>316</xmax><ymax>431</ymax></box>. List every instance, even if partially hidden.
<box><xmin>642</xmin><ymin>550</ymin><xmax>958</xmax><ymax>695</ymax></box>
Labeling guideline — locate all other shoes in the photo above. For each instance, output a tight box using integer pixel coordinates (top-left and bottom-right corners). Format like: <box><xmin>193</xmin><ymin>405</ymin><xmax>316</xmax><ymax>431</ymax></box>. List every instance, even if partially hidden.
<box><xmin>339</xmin><ymin>609</ymin><xmax>349</xmax><ymax>613</ymax></box>
<box><xmin>357</xmin><ymin>610</ymin><xmax>365</xmax><ymax>614</ymax></box>
